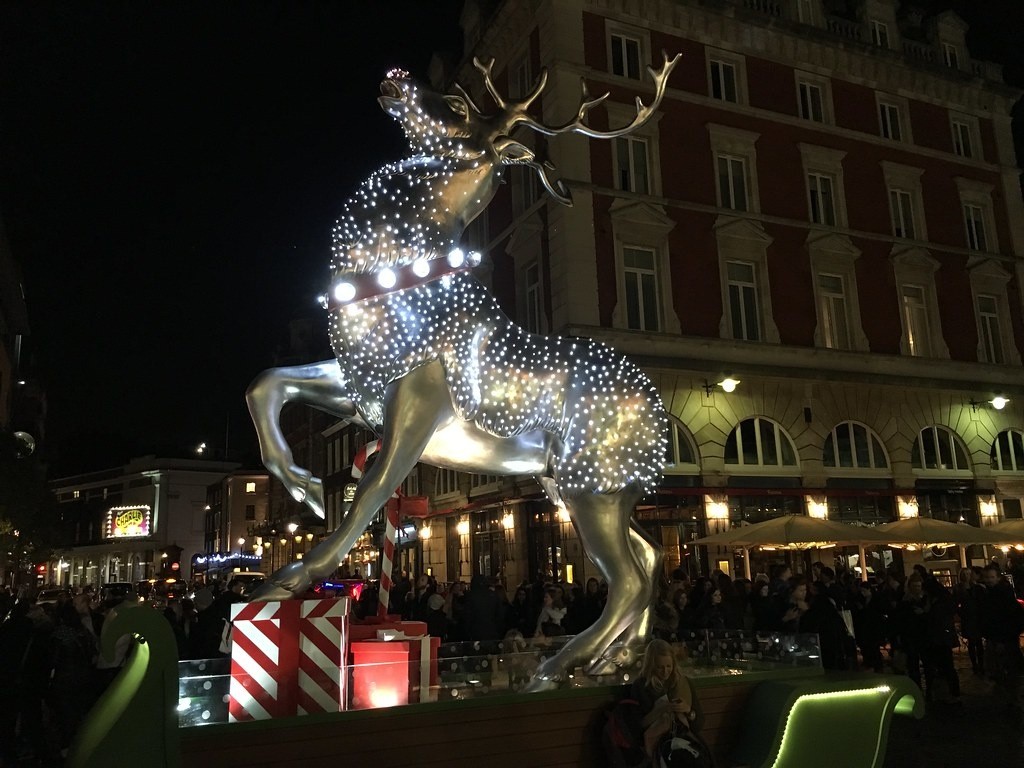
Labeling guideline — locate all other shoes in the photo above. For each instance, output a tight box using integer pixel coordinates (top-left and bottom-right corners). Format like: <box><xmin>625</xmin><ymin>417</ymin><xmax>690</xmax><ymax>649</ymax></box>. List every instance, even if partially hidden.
<box><xmin>947</xmin><ymin>695</ymin><xmax>961</xmax><ymax>703</ymax></box>
<box><xmin>17</xmin><ymin>749</ymin><xmax>35</xmax><ymax>760</ymax></box>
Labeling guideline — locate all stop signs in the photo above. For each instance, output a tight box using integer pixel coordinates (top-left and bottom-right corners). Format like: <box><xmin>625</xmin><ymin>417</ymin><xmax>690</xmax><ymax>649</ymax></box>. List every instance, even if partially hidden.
<box><xmin>171</xmin><ymin>562</ymin><xmax>179</xmax><ymax>571</ymax></box>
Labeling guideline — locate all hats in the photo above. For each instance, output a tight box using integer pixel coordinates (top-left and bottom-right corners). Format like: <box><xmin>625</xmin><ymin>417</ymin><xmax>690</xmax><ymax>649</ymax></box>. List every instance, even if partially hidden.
<box><xmin>428</xmin><ymin>594</ymin><xmax>445</xmax><ymax>611</ymax></box>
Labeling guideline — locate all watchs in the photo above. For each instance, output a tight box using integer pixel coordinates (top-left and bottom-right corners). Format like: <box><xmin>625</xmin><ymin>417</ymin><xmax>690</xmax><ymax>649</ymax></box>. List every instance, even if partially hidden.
<box><xmin>689</xmin><ymin>709</ymin><xmax>696</xmax><ymax>719</ymax></box>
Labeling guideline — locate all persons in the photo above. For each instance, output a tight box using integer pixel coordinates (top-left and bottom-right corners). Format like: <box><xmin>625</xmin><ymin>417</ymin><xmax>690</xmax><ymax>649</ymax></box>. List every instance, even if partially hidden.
<box><xmin>951</xmin><ymin>568</ymin><xmax>987</xmax><ymax>671</ymax></box>
<box><xmin>969</xmin><ymin>566</ymin><xmax>984</xmax><ymax>584</ymax></box>
<box><xmin>977</xmin><ymin>565</ymin><xmax>1024</xmax><ymax>684</ymax></box>
<box><xmin>0</xmin><ymin>560</ymin><xmax>961</xmax><ymax>768</ymax></box>
<box><xmin>627</xmin><ymin>639</ymin><xmax>713</xmax><ymax>768</ymax></box>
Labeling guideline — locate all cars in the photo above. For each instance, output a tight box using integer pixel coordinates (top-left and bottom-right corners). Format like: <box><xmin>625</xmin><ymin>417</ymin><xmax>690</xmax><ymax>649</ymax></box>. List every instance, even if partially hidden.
<box><xmin>37</xmin><ymin>570</ymin><xmax>266</xmax><ymax>610</ymax></box>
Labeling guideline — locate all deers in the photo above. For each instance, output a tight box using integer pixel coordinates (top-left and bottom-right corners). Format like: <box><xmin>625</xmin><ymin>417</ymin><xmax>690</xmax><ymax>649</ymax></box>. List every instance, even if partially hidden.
<box><xmin>243</xmin><ymin>54</ymin><xmax>685</xmax><ymax>694</ymax></box>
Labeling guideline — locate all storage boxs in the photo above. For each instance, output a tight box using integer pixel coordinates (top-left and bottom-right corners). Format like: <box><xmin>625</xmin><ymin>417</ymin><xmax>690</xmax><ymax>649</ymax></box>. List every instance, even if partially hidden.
<box><xmin>227</xmin><ymin>595</ymin><xmax>442</xmax><ymax>722</ymax></box>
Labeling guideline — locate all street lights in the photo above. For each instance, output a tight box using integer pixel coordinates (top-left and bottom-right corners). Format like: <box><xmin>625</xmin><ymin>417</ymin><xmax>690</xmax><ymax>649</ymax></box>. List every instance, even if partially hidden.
<box><xmin>288</xmin><ymin>524</ymin><xmax>299</xmax><ymax>563</ymax></box>
<box><xmin>237</xmin><ymin>538</ymin><xmax>247</xmax><ymax>570</ymax></box>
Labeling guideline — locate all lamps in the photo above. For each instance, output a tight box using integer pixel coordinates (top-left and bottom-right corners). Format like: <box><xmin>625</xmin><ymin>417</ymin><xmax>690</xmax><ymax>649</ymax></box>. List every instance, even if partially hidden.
<box><xmin>705</xmin><ymin>374</ymin><xmax>741</xmax><ymax>397</ymax></box>
<box><xmin>969</xmin><ymin>395</ymin><xmax>1010</xmax><ymax>413</ymax></box>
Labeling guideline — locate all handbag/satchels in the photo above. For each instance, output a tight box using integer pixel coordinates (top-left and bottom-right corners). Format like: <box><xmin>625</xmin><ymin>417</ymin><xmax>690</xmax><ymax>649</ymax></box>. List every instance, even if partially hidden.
<box><xmin>601</xmin><ymin>699</ymin><xmax>644</xmax><ymax>768</ymax></box>
<box><xmin>829</xmin><ymin>596</ymin><xmax>855</xmax><ymax>639</ymax></box>
<box><xmin>218</xmin><ymin>617</ymin><xmax>233</xmax><ymax>654</ymax></box>
<box><xmin>541</xmin><ymin>617</ymin><xmax>565</xmax><ymax>637</ymax></box>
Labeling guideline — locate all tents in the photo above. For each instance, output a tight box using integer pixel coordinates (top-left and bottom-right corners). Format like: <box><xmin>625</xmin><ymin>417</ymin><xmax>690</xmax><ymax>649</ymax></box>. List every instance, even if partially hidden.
<box><xmin>794</xmin><ymin>514</ymin><xmax>925</xmax><ymax>582</ymax></box>
<box><xmin>684</xmin><ymin>514</ymin><xmax>838</xmax><ymax>581</ymax></box>
<box><xmin>863</xmin><ymin>516</ymin><xmax>1022</xmax><ymax>581</ymax></box>
